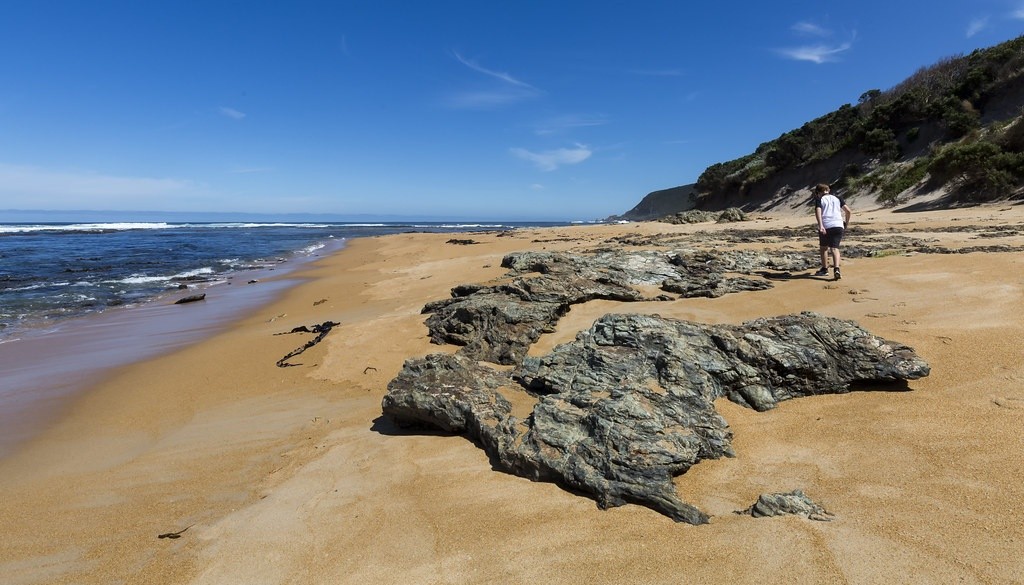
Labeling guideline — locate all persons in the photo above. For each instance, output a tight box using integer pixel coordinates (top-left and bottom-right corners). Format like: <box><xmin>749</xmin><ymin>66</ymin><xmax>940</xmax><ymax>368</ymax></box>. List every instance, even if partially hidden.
<box><xmin>815</xmin><ymin>183</ymin><xmax>850</xmax><ymax>280</ymax></box>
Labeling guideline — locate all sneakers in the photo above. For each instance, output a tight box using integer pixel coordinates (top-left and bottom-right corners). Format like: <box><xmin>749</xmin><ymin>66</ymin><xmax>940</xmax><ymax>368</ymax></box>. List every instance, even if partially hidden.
<box><xmin>815</xmin><ymin>269</ymin><xmax>828</xmax><ymax>277</ymax></box>
<box><xmin>833</xmin><ymin>268</ymin><xmax>841</xmax><ymax>280</ymax></box>
